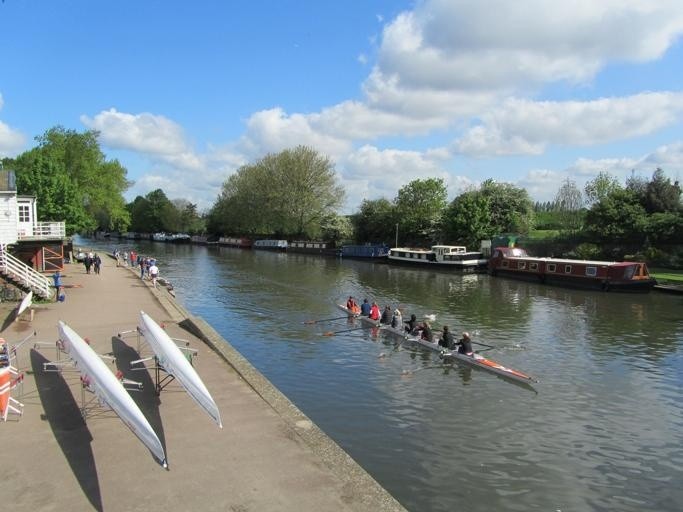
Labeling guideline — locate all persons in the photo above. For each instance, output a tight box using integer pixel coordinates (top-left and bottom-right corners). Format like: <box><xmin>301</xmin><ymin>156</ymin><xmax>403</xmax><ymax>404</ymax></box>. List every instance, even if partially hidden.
<box><xmin>380</xmin><ymin>304</ymin><xmax>392</xmax><ymax>325</ymax></box>
<box><xmin>347</xmin><ymin>296</ymin><xmax>359</xmax><ymax>310</ymax></box>
<box><xmin>369</xmin><ymin>301</ymin><xmax>381</xmax><ymax>320</ymax></box>
<box><xmin>391</xmin><ymin>309</ymin><xmax>402</xmax><ymax>329</ymax></box>
<box><xmin>74</xmin><ymin>248</ymin><xmax>159</xmax><ymax>287</ymax></box>
<box><xmin>361</xmin><ymin>298</ymin><xmax>371</xmax><ymax>316</ymax></box>
<box><xmin>415</xmin><ymin>322</ymin><xmax>432</xmax><ymax>342</ymax></box>
<box><xmin>438</xmin><ymin>326</ymin><xmax>456</xmax><ymax>350</ymax></box>
<box><xmin>53</xmin><ymin>271</ymin><xmax>62</xmax><ymax>301</ymax></box>
<box><xmin>404</xmin><ymin>314</ymin><xmax>419</xmax><ymax>336</ymax></box>
<box><xmin>454</xmin><ymin>332</ymin><xmax>473</xmax><ymax>356</ymax></box>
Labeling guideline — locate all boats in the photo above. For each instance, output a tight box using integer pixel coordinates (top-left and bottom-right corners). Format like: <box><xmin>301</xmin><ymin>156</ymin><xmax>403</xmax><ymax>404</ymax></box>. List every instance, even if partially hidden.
<box><xmin>330</xmin><ymin>300</ymin><xmax>541</xmax><ymax>387</ymax></box>
<box><xmin>57</xmin><ymin>319</ymin><xmax>169</xmax><ymax>472</ymax></box>
<box><xmin>120</xmin><ymin>231</ymin><xmax>209</xmax><ymax>245</ymax></box>
<box><xmin>217</xmin><ymin>236</ymin><xmax>330</xmax><ymax>256</ymax></box>
<box><xmin>17</xmin><ymin>290</ymin><xmax>33</xmax><ymax>316</ymax></box>
<box><xmin>156</xmin><ymin>276</ymin><xmax>175</xmax><ymax>298</ymax></box>
<box><xmin>136</xmin><ymin>309</ymin><xmax>223</xmax><ymax>430</ymax></box>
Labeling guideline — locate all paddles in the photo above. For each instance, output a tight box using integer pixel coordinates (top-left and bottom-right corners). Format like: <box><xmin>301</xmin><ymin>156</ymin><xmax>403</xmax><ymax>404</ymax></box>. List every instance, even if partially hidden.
<box><xmin>304</xmin><ymin>315</ymin><xmax>365</xmax><ymax>324</ymax></box>
<box><xmin>323</xmin><ymin>323</ymin><xmax>385</xmax><ymax>336</ymax></box>
